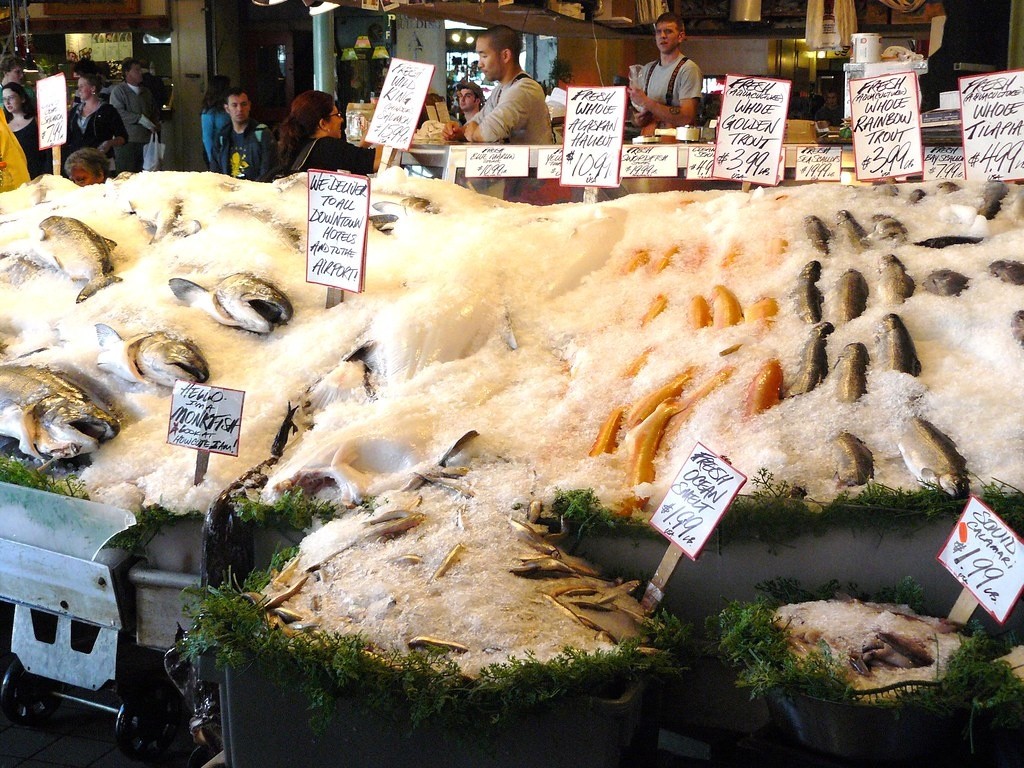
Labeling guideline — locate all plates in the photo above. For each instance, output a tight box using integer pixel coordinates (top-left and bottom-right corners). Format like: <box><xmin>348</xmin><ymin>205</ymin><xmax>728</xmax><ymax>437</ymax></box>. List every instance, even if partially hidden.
<box><xmin>93</xmin><ymin>32</ymin><xmax>132</xmax><ymax>44</ymax></box>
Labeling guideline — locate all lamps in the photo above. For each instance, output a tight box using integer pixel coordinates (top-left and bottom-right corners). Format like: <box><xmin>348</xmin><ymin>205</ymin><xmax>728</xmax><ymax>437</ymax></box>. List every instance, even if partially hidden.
<box><xmin>450</xmin><ymin>30</ymin><xmax>463</xmax><ymax>44</ymax></box>
<box><xmin>465</xmin><ymin>30</ymin><xmax>475</xmax><ymax>44</ymax></box>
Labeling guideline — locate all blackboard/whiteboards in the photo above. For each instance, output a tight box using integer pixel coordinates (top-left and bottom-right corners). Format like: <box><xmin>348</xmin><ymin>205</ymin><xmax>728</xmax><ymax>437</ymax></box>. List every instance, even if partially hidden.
<box><xmin>958</xmin><ymin>67</ymin><xmax>1024</xmax><ymax>185</ymax></box>
<box><xmin>560</xmin><ymin>85</ymin><xmax>629</xmax><ymax>187</ymax></box>
<box><xmin>35</xmin><ymin>70</ymin><xmax>69</xmax><ymax>149</ymax></box>
<box><xmin>795</xmin><ymin>146</ymin><xmax>843</xmax><ymax>183</ymax></box>
<box><xmin>537</xmin><ymin>146</ymin><xmax>679</xmax><ymax>179</ymax></box>
<box><xmin>306</xmin><ymin>168</ymin><xmax>370</xmax><ymax>294</ymax></box>
<box><xmin>165</xmin><ymin>379</ymin><xmax>246</xmax><ymax>456</ymax></box>
<box><xmin>364</xmin><ymin>56</ymin><xmax>435</xmax><ymax>152</ymax></box>
<box><xmin>850</xmin><ymin>72</ymin><xmax>925</xmax><ymax>182</ymax></box>
<box><xmin>687</xmin><ymin>145</ymin><xmax>786</xmax><ymax>184</ymax></box>
<box><xmin>465</xmin><ymin>145</ymin><xmax>530</xmax><ymax>178</ymax></box>
<box><xmin>922</xmin><ymin>144</ymin><xmax>965</xmax><ymax>181</ymax></box>
<box><xmin>935</xmin><ymin>493</ymin><xmax>1024</xmax><ymax>627</ymax></box>
<box><xmin>712</xmin><ymin>73</ymin><xmax>791</xmax><ymax>186</ymax></box>
<box><xmin>649</xmin><ymin>440</ymin><xmax>747</xmax><ymax>561</ymax></box>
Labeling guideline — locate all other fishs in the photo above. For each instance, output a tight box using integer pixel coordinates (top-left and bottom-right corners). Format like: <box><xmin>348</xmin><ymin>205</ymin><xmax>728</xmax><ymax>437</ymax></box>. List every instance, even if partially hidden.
<box><xmin>0</xmin><ymin>169</ymin><xmax>1024</xmax><ymax>688</ymax></box>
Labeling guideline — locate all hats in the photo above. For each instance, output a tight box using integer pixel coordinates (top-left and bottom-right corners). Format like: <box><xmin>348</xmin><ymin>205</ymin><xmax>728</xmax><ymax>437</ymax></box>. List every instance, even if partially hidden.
<box><xmin>457</xmin><ymin>82</ymin><xmax>483</xmax><ymax>101</ymax></box>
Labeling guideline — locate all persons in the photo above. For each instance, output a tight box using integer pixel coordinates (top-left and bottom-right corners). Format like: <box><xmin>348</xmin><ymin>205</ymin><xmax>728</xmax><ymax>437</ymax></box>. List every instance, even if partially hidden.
<box><xmin>73</xmin><ymin>53</ymin><xmax>108</xmax><ymax>79</ymax></box>
<box><xmin>62</xmin><ymin>51</ymin><xmax>77</xmax><ymax>65</ymax></box>
<box><xmin>109</xmin><ymin>56</ymin><xmax>161</xmax><ymax>177</ymax></box>
<box><xmin>626</xmin><ymin>12</ymin><xmax>703</xmax><ymax>136</ymax></box>
<box><xmin>2</xmin><ymin>81</ymin><xmax>39</xmax><ymax>180</ymax></box>
<box><xmin>64</xmin><ymin>147</ymin><xmax>109</xmax><ymax>188</ymax></box>
<box><xmin>791</xmin><ymin>90</ymin><xmax>843</xmax><ymax>126</ymax></box>
<box><xmin>0</xmin><ymin>55</ymin><xmax>34</xmax><ymax>108</ymax></box>
<box><xmin>92</xmin><ymin>32</ymin><xmax>132</xmax><ymax>43</ymax></box>
<box><xmin>67</xmin><ymin>71</ymin><xmax>129</xmax><ymax>179</ymax></box>
<box><xmin>455</xmin><ymin>81</ymin><xmax>485</xmax><ymax>126</ymax></box>
<box><xmin>200</xmin><ymin>72</ymin><xmax>232</xmax><ymax>173</ymax></box>
<box><xmin>442</xmin><ymin>24</ymin><xmax>572</xmax><ymax>206</ymax></box>
<box><xmin>25</xmin><ymin>52</ymin><xmax>36</xmax><ymax>70</ymax></box>
<box><xmin>209</xmin><ymin>86</ymin><xmax>281</xmax><ymax>181</ymax></box>
<box><xmin>278</xmin><ymin>90</ymin><xmax>382</xmax><ymax>176</ymax></box>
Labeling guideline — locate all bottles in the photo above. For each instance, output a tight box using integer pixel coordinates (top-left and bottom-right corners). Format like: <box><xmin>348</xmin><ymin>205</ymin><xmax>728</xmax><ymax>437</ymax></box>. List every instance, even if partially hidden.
<box><xmin>150</xmin><ymin>61</ymin><xmax>155</xmax><ymax>76</ymax></box>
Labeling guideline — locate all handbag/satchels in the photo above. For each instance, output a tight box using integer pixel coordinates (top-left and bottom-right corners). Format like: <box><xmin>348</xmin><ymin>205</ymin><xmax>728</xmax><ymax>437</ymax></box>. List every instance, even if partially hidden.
<box><xmin>143</xmin><ymin>131</ymin><xmax>166</xmax><ymax>172</ymax></box>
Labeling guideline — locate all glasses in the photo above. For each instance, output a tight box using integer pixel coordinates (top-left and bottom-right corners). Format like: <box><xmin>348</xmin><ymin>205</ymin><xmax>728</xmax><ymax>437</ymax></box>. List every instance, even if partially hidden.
<box><xmin>328</xmin><ymin>111</ymin><xmax>342</xmax><ymax>118</ymax></box>
<box><xmin>1</xmin><ymin>94</ymin><xmax>19</xmax><ymax>102</ymax></box>
